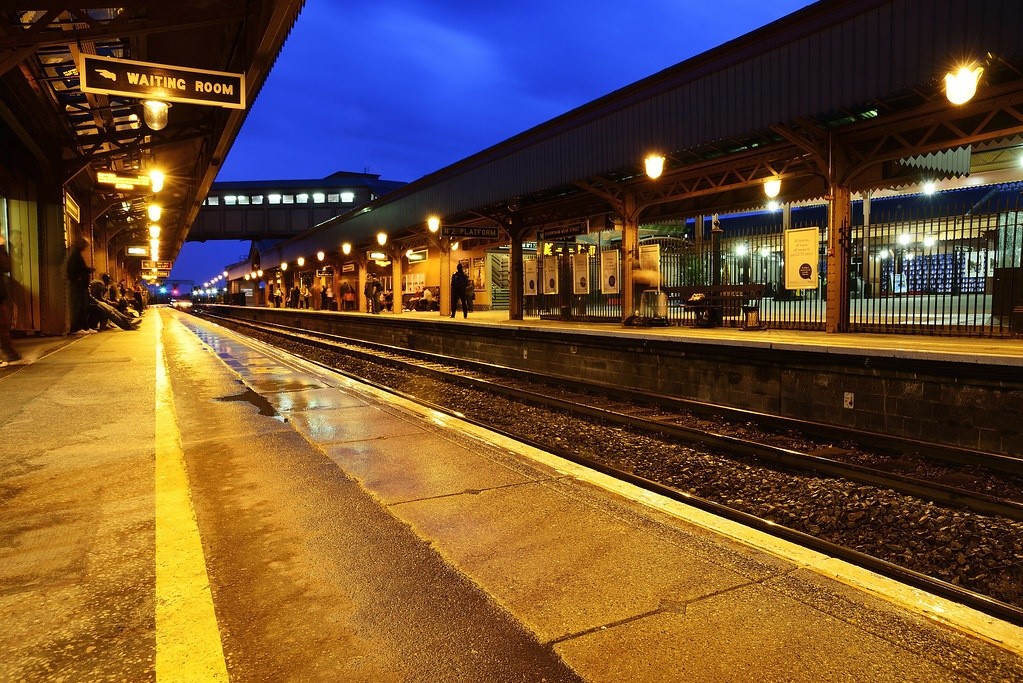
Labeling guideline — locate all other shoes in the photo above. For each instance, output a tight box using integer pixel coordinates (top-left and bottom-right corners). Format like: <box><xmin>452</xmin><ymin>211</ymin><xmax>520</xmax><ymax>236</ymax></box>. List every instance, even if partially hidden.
<box><xmin>126</xmin><ymin>325</ymin><xmax>140</xmax><ymax>330</ymax></box>
<box><xmin>372</xmin><ymin>311</ymin><xmax>380</xmax><ymax>314</ymax></box>
<box><xmin>382</xmin><ymin>309</ymin><xmax>388</xmax><ymax>312</ymax></box>
<box><xmin>129</xmin><ymin>318</ymin><xmax>143</xmax><ymax>327</ymax></box>
<box><xmin>411</xmin><ymin>309</ymin><xmax>416</xmax><ymax>311</ymax></box>
<box><xmin>467</xmin><ymin>309</ymin><xmax>473</xmax><ymax>313</ymax></box>
<box><xmin>464</xmin><ymin>316</ymin><xmax>467</xmax><ymax>318</ymax></box>
<box><xmin>450</xmin><ymin>316</ymin><xmax>454</xmax><ymax>318</ymax></box>
<box><xmin>404</xmin><ymin>309</ymin><xmax>410</xmax><ymax>311</ymax></box>
<box><xmin>94</xmin><ymin>324</ymin><xmax>117</xmax><ymax>331</ymax></box>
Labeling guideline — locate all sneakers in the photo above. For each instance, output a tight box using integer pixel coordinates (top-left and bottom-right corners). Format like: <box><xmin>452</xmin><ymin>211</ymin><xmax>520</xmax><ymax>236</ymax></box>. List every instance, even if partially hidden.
<box><xmin>9</xmin><ymin>356</ymin><xmax>30</xmax><ymax>365</ymax></box>
<box><xmin>73</xmin><ymin>329</ymin><xmax>89</xmax><ymax>335</ymax></box>
<box><xmin>87</xmin><ymin>328</ymin><xmax>98</xmax><ymax>334</ymax></box>
<box><xmin>0</xmin><ymin>360</ymin><xmax>8</xmax><ymax>367</ymax></box>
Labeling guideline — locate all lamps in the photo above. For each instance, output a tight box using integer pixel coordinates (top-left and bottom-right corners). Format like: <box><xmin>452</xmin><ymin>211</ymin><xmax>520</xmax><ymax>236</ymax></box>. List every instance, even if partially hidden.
<box><xmin>137</xmin><ymin>98</ymin><xmax>172</xmax><ymax>130</ymax></box>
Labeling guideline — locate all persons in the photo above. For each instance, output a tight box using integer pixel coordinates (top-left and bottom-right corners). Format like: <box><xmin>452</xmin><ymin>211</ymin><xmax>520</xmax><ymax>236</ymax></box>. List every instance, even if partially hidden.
<box><xmin>64</xmin><ymin>233</ymin><xmax>98</xmax><ymax>337</ymax></box>
<box><xmin>450</xmin><ymin>262</ymin><xmax>470</xmax><ymax>318</ymax></box>
<box><xmin>93</xmin><ymin>272</ymin><xmax>149</xmax><ymax>332</ymax></box>
<box><xmin>465</xmin><ymin>279</ymin><xmax>475</xmax><ymax>312</ymax></box>
<box><xmin>0</xmin><ymin>218</ymin><xmax>22</xmax><ymax>368</ymax></box>
<box><xmin>275</xmin><ymin>271</ymin><xmax>440</xmax><ymax>315</ymax></box>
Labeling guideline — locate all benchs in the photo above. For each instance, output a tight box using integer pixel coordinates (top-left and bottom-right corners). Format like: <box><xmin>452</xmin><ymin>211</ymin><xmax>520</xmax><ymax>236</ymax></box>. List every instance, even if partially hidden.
<box><xmin>675</xmin><ymin>284</ymin><xmax>766</xmax><ymax>330</ymax></box>
